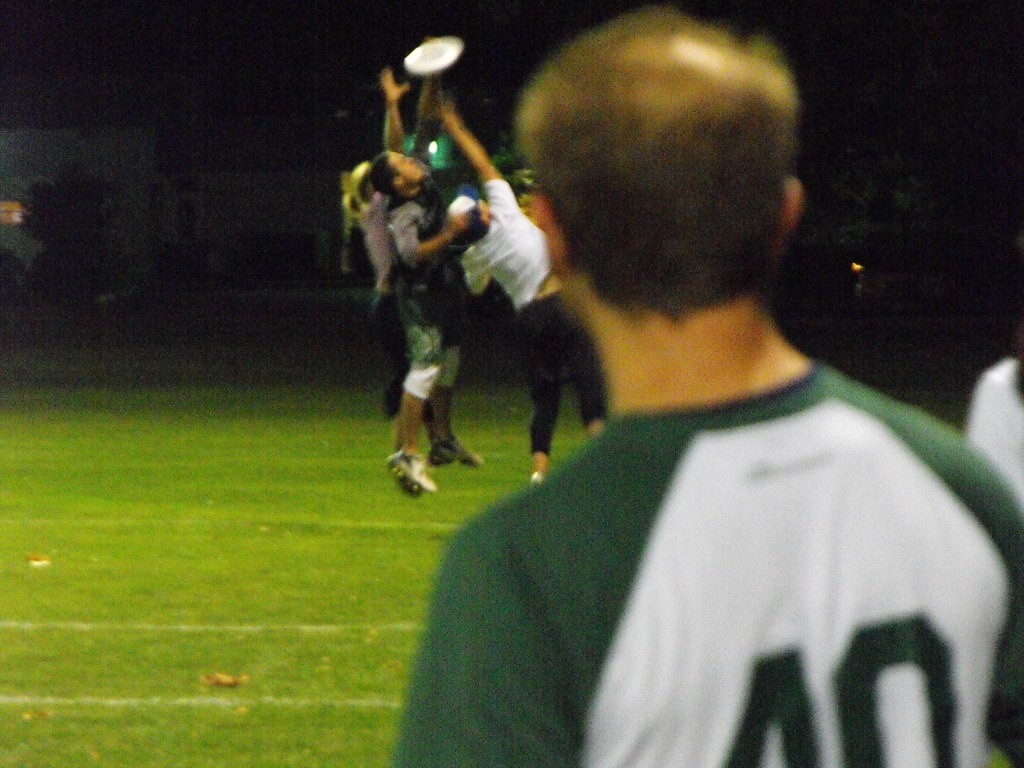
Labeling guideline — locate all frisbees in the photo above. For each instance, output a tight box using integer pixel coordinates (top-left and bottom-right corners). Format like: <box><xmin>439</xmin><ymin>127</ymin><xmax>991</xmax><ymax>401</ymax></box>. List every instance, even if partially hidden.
<box><xmin>404</xmin><ymin>36</ymin><xmax>464</xmax><ymax>75</ymax></box>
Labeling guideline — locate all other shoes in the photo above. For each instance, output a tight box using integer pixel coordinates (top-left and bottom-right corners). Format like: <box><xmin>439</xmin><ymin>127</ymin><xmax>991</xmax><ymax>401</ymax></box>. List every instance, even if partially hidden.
<box><xmin>530</xmin><ymin>473</ymin><xmax>543</xmax><ymax>489</ymax></box>
<box><xmin>385</xmin><ymin>449</ymin><xmax>438</xmax><ymax>493</ymax></box>
<box><xmin>427</xmin><ymin>441</ymin><xmax>485</xmax><ymax>467</ymax></box>
<box><xmin>386</xmin><ymin>455</ymin><xmax>422</xmax><ymax>498</ymax></box>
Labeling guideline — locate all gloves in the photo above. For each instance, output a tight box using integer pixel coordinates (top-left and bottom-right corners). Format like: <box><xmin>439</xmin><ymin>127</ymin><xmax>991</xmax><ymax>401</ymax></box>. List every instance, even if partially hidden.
<box><xmin>445</xmin><ymin>202</ymin><xmax>491</xmax><ymax>246</ymax></box>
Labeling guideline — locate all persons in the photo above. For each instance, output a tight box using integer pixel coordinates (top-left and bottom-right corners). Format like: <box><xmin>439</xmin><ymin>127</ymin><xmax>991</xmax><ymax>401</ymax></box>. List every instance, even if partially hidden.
<box><xmin>339</xmin><ymin>69</ymin><xmax>607</xmax><ymax>497</ymax></box>
<box><xmin>963</xmin><ymin>355</ymin><xmax>1024</xmax><ymax>492</ymax></box>
<box><xmin>384</xmin><ymin>23</ymin><xmax>1024</xmax><ymax>768</ymax></box>
